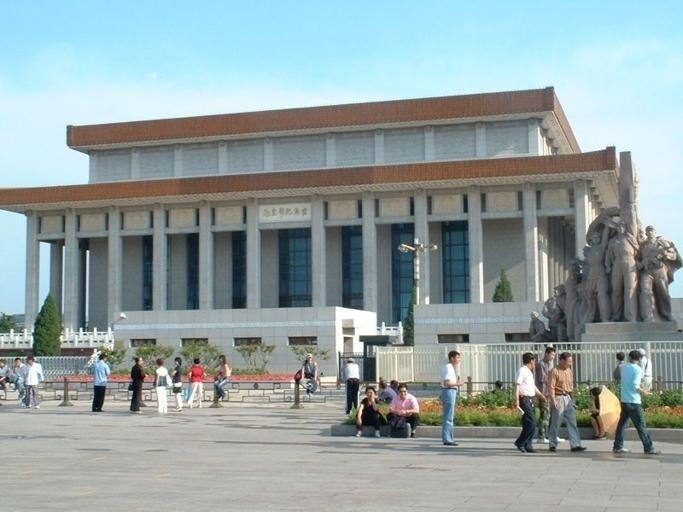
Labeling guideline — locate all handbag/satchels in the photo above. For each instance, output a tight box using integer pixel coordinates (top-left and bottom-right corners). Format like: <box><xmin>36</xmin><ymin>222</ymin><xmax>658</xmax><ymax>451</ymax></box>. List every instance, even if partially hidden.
<box><xmin>167</xmin><ymin>375</ymin><xmax>173</xmax><ymax>389</ymax></box>
<box><xmin>294</xmin><ymin>369</ymin><xmax>306</xmax><ymax>381</ymax></box>
<box><xmin>172</xmin><ymin>386</ymin><xmax>182</xmax><ymax>393</ymax></box>
<box><xmin>391</xmin><ymin>423</ymin><xmax>411</xmax><ymax>437</ymax></box>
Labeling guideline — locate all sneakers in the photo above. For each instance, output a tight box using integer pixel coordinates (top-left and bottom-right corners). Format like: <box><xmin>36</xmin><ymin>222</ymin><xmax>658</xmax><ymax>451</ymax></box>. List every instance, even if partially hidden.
<box><xmin>515</xmin><ymin>441</ymin><xmax>535</xmax><ymax>453</ymax></box>
<box><xmin>644</xmin><ymin>449</ymin><xmax>660</xmax><ymax>453</ymax></box>
<box><xmin>444</xmin><ymin>442</ymin><xmax>460</xmax><ymax>445</ymax></box>
<box><xmin>572</xmin><ymin>447</ymin><xmax>586</xmax><ymax>452</ymax></box>
<box><xmin>356</xmin><ymin>431</ymin><xmax>362</xmax><ymax>437</ymax></box>
<box><xmin>375</xmin><ymin>430</ymin><xmax>380</xmax><ymax>437</ymax></box>
<box><xmin>615</xmin><ymin>448</ymin><xmax>629</xmax><ymax>452</ymax></box>
<box><xmin>549</xmin><ymin>448</ymin><xmax>556</xmax><ymax>452</ymax></box>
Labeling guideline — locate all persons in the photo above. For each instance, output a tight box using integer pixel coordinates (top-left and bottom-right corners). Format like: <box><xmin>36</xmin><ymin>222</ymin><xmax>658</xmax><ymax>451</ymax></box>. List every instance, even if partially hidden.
<box><xmin>638</xmin><ymin>225</ymin><xmax>677</xmax><ymax>322</ymax></box>
<box><xmin>530</xmin><ymin>260</ymin><xmax>583</xmax><ymax>342</ymax></box>
<box><xmin>300</xmin><ymin>353</ymin><xmax>320</xmax><ymax>394</ymax></box>
<box><xmin>343</xmin><ymin>358</ymin><xmax>360</xmax><ymax>415</ymax></box>
<box><xmin>214</xmin><ymin>355</ymin><xmax>231</xmax><ymax>401</ymax></box>
<box><xmin>604</xmin><ymin>221</ymin><xmax>637</xmax><ymax>322</ymax></box>
<box><xmin>187</xmin><ymin>358</ymin><xmax>206</xmax><ymax>409</ymax></box>
<box><xmin>440</xmin><ymin>350</ymin><xmax>464</xmax><ymax>447</ymax></box>
<box><xmin>171</xmin><ymin>358</ymin><xmax>183</xmax><ymax>411</ymax></box>
<box><xmin>1</xmin><ymin>356</ymin><xmax>45</xmax><ymax>409</ymax></box>
<box><xmin>153</xmin><ymin>358</ymin><xmax>172</xmax><ymax>415</ymax></box>
<box><xmin>514</xmin><ymin>347</ymin><xmax>661</xmax><ymax>455</ymax></box>
<box><xmin>583</xmin><ymin>224</ymin><xmax>611</xmax><ymax>324</ymax></box>
<box><xmin>130</xmin><ymin>357</ymin><xmax>148</xmax><ymax>413</ymax></box>
<box><xmin>91</xmin><ymin>354</ymin><xmax>110</xmax><ymax>412</ymax></box>
<box><xmin>355</xmin><ymin>379</ymin><xmax>420</xmax><ymax>440</ymax></box>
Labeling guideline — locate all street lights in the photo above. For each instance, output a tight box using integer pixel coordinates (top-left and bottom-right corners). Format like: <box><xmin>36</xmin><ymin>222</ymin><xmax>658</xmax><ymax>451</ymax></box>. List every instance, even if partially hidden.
<box><xmin>396</xmin><ymin>238</ymin><xmax>440</xmax><ymax>305</ymax></box>
